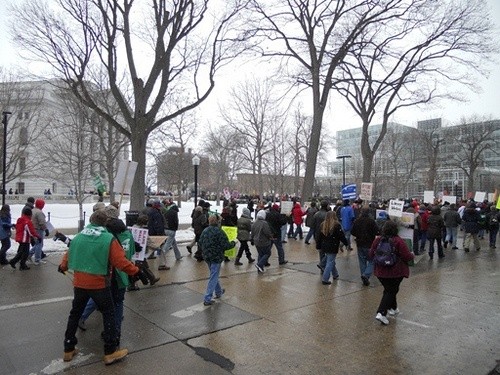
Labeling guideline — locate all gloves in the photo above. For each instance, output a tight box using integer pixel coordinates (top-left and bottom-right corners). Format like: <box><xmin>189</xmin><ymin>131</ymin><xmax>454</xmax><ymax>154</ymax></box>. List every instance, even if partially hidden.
<box><xmin>45</xmin><ymin>230</ymin><xmax>49</xmax><ymax>237</ymax></box>
<box><xmin>137</xmin><ymin>271</ymin><xmax>148</xmax><ymax>286</ymax></box>
<box><xmin>52</xmin><ymin>232</ymin><xmax>67</xmax><ymax>243</ymax></box>
<box><xmin>38</xmin><ymin>238</ymin><xmax>42</xmax><ymax>243</ymax></box>
<box><xmin>58</xmin><ymin>264</ymin><xmax>65</xmax><ymax>275</ymax></box>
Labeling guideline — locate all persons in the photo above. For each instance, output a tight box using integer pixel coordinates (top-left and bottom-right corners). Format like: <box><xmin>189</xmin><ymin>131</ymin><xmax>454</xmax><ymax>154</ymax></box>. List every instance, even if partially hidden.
<box><xmin>44</xmin><ymin>188</ymin><xmax>51</xmax><ymax>194</ymax></box>
<box><xmin>9</xmin><ymin>188</ymin><xmax>18</xmax><ymax>194</ymax></box>
<box><xmin>281</xmin><ymin>193</ymin><xmax>292</xmax><ymax>201</ymax></box>
<box><xmin>0</xmin><ymin>197</ymin><xmax>49</xmax><ymax>271</ymax></box>
<box><xmin>57</xmin><ymin>210</ymin><xmax>139</xmax><ymax>365</ymax></box>
<box><xmin>281</xmin><ymin>201</ymin><xmax>319</xmax><ymax>245</ymax></box>
<box><xmin>55</xmin><ymin>189</ymin><xmax>160</xmax><ymax>337</ymax></box>
<box><xmin>251</xmin><ymin>209</ymin><xmax>273</xmax><ymax>273</ymax></box>
<box><xmin>311</xmin><ymin>200</ymin><xmax>349</xmax><ymax>285</ymax></box>
<box><xmin>334</xmin><ymin>197</ymin><xmax>379</xmax><ymax>286</ymax></box>
<box><xmin>369</xmin><ymin>221</ymin><xmax>415</xmax><ymax>325</ymax></box>
<box><xmin>247</xmin><ymin>198</ymin><xmax>271</xmax><ymax>219</ymax></box>
<box><xmin>265</xmin><ymin>204</ymin><xmax>291</xmax><ymax>266</ymax></box>
<box><xmin>220</xmin><ymin>196</ymin><xmax>239</xmax><ymax>263</ymax></box>
<box><xmin>141</xmin><ymin>197</ymin><xmax>185</xmax><ymax>270</ymax></box>
<box><xmin>186</xmin><ymin>199</ymin><xmax>211</xmax><ymax>255</ymax></box>
<box><xmin>234</xmin><ymin>208</ymin><xmax>255</xmax><ymax>265</ymax></box>
<box><xmin>199</xmin><ymin>214</ymin><xmax>239</xmax><ymax>306</ymax></box>
<box><xmin>440</xmin><ymin>198</ymin><xmax>500</xmax><ymax>252</ymax></box>
<box><xmin>377</xmin><ymin>197</ymin><xmax>445</xmax><ymax>259</ymax></box>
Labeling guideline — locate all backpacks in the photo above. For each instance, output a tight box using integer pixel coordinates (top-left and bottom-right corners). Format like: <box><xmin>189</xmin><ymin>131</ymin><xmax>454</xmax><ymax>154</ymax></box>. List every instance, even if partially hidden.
<box><xmin>374</xmin><ymin>234</ymin><xmax>399</xmax><ymax>267</ymax></box>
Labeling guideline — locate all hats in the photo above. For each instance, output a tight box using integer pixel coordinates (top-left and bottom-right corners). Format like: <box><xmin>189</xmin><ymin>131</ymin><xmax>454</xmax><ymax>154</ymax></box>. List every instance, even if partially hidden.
<box><xmin>90</xmin><ymin>201</ymin><xmax>120</xmax><ymax>227</ymax></box>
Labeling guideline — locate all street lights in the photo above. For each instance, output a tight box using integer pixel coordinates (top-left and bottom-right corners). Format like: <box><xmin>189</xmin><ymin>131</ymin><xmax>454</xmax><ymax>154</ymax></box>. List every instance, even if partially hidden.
<box><xmin>1</xmin><ymin>111</ymin><xmax>12</xmax><ymax>207</ymax></box>
<box><xmin>192</xmin><ymin>153</ymin><xmax>201</xmax><ymax>207</ymax></box>
<box><xmin>336</xmin><ymin>155</ymin><xmax>352</xmax><ymax>186</ymax></box>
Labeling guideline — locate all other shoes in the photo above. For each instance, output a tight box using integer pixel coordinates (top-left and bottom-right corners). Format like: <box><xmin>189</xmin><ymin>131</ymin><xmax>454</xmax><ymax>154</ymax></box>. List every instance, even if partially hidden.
<box><xmin>186</xmin><ymin>246</ymin><xmax>192</xmax><ymax>254</ymax></box>
<box><xmin>256</xmin><ymin>263</ymin><xmax>265</xmax><ymax>272</ymax></box>
<box><xmin>283</xmin><ymin>232</ymin><xmax>369</xmax><ymax>287</ymax></box>
<box><xmin>79</xmin><ymin>320</ymin><xmax>87</xmax><ymax>332</ymax></box>
<box><xmin>388</xmin><ymin>307</ymin><xmax>400</xmax><ymax>315</ymax></box>
<box><xmin>0</xmin><ymin>252</ymin><xmax>47</xmax><ymax>270</ymax></box>
<box><xmin>64</xmin><ymin>345</ymin><xmax>81</xmax><ymax>361</ymax></box>
<box><xmin>249</xmin><ymin>259</ymin><xmax>255</xmax><ymax>264</ymax></box>
<box><xmin>215</xmin><ymin>289</ymin><xmax>225</xmax><ymax>298</ymax></box>
<box><xmin>235</xmin><ymin>261</ymin><xmax>243</xmax><ymax>266</ymax></box>
<box><xmin>103</xmin><ymin>349</ymin><xmax>129</xmax><ymax>364</ymax></box>
<box><xmin>127</xmin><ymin>256</ymin><xmax>184</xmax><ymax>292</ymax></box>
<box><xmin>375</xmin><ymin>313</ymin><xmax>389</xmax><ymax>325</ymax></box>
<box><xmin>263</xmin><ymin>262</ymin><xmax>271</xmax><ymax>266</ymax></box>
<box><xmin>414</xmin><ymin>239</ymin><xmax>497</xmax><ymax>259</ymax></box>
<box><xmin>204</xmin><ymin>301</ymin><xmax>215</xmax><ymax>305</ymax></box>
<box><xmin>280</xmin><ymin>260</ymin><xmax>288</xmax><ymax>265</ymax></box>
